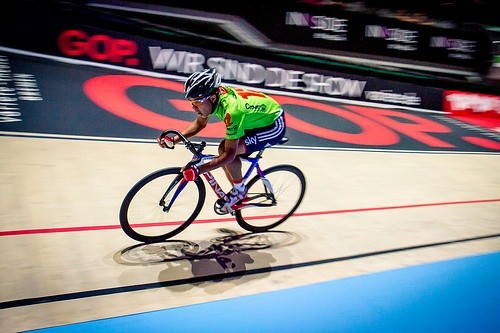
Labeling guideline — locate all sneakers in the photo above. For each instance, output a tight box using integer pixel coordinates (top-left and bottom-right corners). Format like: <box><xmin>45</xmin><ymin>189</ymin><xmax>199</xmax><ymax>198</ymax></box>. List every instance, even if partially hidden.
<box><xmin>217</xmin><ymin>184</ymin><xmax>248</xmax><ymax>209</ymax></box>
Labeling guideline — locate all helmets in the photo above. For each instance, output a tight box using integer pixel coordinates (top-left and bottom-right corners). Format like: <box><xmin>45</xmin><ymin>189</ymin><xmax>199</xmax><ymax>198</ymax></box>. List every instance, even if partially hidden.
<box><xmin>183</xmin><ymin>66</ymin><xmax>222</xmax><ymax>103</ymax></box>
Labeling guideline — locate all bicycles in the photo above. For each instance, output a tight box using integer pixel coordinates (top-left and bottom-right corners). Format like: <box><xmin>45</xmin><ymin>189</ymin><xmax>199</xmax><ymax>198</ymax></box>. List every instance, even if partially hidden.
<box><xmin>119</xmin><ymin>128</ymin><xmax>308</xmax><ymax>242</ymax></box>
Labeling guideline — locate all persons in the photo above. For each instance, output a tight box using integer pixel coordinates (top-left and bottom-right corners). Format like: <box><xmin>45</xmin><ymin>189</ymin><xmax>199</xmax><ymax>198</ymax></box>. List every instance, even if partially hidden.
<box><xmin>157</xmin><ymin>66</ymin><xmax>287</xmax><ymax>207</ymax></box>
